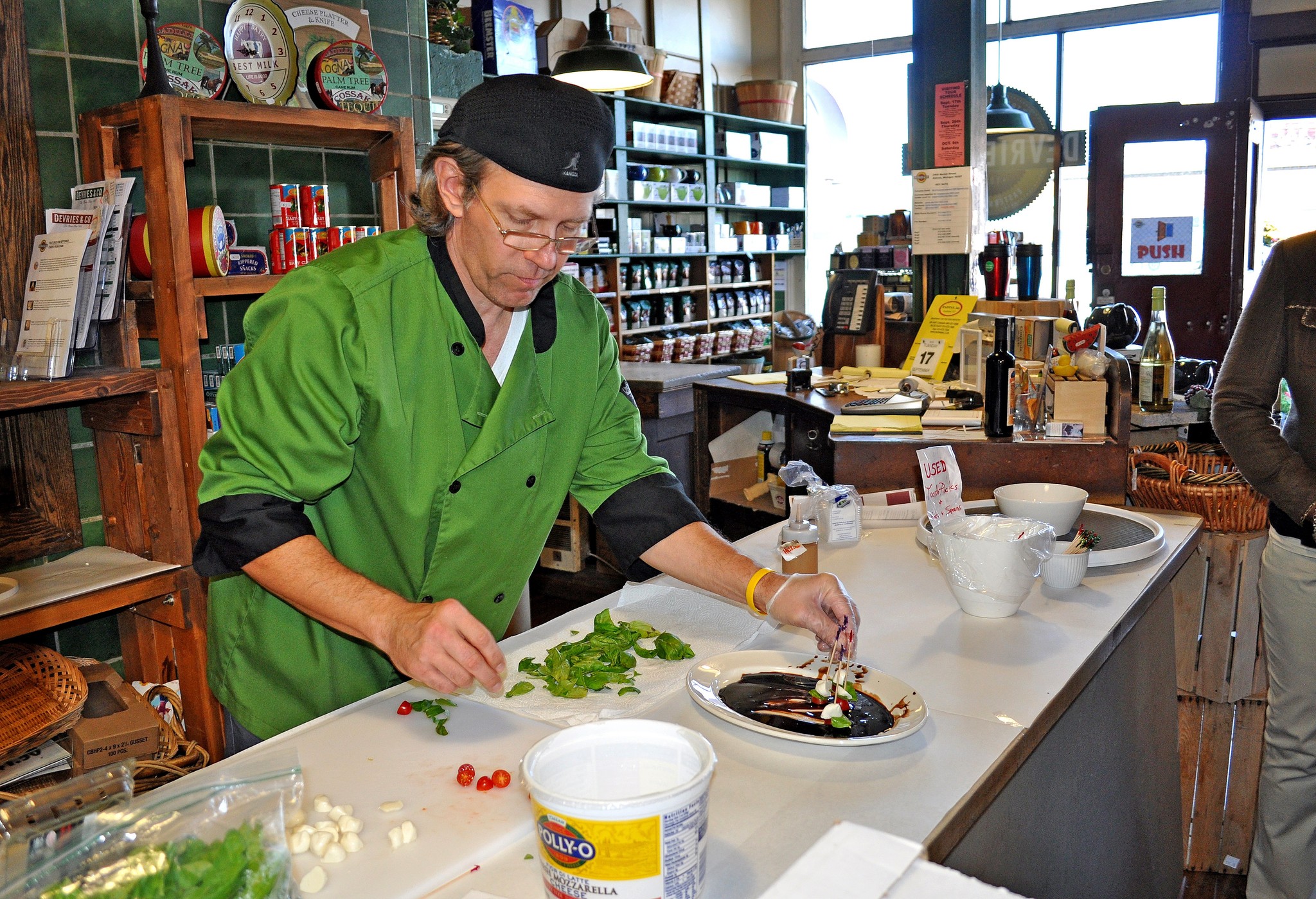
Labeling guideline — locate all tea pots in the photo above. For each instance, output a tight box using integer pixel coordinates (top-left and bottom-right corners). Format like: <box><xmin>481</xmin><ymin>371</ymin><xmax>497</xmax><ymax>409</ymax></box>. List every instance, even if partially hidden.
<box><xmin>1084</xmin><ymin>302</ymin><xmax>1141</xmax><ymax>350</ymax></box>
<box><xmin>1174</xmin><ymin>352</ymin><xmax>1218</xmax><ymax>395</ymax></box>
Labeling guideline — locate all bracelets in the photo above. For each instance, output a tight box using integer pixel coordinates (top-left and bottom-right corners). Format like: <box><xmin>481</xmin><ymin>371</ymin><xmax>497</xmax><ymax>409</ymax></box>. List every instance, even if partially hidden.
<box><xmin>1312</xmin><ymin>508</ymin><xmax>1316</xmax><ymax>545</ymax></box>
<box><xmin>746</xmin><ymin>565</ymin><xmax>781</xmax><ymax>622</ymax></box>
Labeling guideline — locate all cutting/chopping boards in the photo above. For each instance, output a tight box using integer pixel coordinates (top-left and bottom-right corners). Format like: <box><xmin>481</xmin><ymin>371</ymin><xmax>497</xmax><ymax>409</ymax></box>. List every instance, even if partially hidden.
<box><xmin>95</xmin><ymin>665</ymin><xmax>584</xmax><ymax>899</ymax></box>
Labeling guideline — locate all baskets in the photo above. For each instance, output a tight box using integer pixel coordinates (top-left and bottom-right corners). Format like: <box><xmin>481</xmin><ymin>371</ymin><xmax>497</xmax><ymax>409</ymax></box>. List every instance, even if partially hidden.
<box><xmin>0</xmin><ymin>642</ymin><xmax>89</xmax><ymax>769</ymax></box>
<box><xmin>1127</xmin><ymin>442</ymin><xmax>1272</xmax><ymax>533</ymax></box>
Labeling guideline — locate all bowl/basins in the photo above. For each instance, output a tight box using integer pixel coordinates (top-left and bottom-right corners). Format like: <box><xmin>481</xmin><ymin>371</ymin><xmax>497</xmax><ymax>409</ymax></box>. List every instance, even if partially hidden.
<box><xmin>1042</xmin><ymin>541</ymin><xmax>1091</xmax><ymax>589</ymax></box>
<box><xmin>993</xmin><ymin>482</ymin><xmax>1090</xmax><ymax>536</ymax></box>
<box><xmin>934</xmin><ymin>517</ymin><xmax>1055</xmax><ymax>619</ymax></box>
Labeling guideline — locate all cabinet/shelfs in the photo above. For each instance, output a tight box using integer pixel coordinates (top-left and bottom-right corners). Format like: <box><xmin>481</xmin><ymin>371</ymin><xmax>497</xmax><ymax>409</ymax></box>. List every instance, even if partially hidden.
<box><xmin>537</xmin><ymin>81</ymin><xmax>814</xmax><ymax>386</ymax></box>
<box><xmin>0</xmin><ymin>0</ymin><xmax>444</xmax><ymax>841</ymax></box>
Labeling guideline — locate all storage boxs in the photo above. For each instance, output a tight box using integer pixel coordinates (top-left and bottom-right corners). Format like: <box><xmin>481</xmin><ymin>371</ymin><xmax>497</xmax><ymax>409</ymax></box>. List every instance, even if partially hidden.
<box><xmin>467</xmin><ymin>0</ymin><xmax>541</xmax><ymax>81</ymax></box>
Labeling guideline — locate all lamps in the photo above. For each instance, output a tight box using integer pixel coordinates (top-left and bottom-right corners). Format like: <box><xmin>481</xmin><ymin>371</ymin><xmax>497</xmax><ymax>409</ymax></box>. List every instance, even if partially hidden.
<box><xmin>979</xmin><ymin>0</ymin><xmax>1037</xmax><ymax>134</ymax></box>
<box><xmin>543</xmin><ymin>0</ymin><xmax>657</xmax><ymax>94</ymax></box>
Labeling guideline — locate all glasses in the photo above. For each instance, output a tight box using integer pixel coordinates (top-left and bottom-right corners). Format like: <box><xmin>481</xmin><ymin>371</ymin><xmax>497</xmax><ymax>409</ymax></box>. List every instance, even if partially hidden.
<box><xmin>462</xmin><ymin>176</ymin><xmax>599</xmax><ymax>255</ymax></box>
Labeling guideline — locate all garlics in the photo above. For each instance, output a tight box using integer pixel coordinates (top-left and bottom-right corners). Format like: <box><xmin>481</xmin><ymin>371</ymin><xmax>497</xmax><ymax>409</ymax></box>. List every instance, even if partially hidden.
<box><xmin>285</xmin><ymin>793</ymin><xmax>419</xmax><ymax>892</ymax></box>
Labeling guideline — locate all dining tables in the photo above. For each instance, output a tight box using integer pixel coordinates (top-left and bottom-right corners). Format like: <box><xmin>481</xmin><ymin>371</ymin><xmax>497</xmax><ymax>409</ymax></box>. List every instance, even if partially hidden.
<box><xmin>82</xmin><ymin>489</ymin><xmax>1213</xmax><ymax>899</ymax></box>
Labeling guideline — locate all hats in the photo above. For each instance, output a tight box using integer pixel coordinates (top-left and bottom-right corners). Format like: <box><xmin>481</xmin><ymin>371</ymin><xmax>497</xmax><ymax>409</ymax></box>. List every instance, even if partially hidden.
<box><xmin>438</xmin><ymin>74</ymin><xmax>617</xmax><ymax>192</ymax></box>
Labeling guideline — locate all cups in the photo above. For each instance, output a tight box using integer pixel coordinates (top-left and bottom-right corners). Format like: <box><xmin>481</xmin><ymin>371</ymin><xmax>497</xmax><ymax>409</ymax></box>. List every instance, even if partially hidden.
<box><xmin>980</xmin><ymin>243</ymin><xmax>1013</xmax><ymax>300</ymax></box>
<box><xmin>657</xmin><ymin>220</ymin><xmax>792</xmax><ymax>251</ymax></box>
<box><xmin>1012</xmin><ymin>395</ymin><xmax>1048</xmax><ymax>443</ymax></box>
<box><xmin>626</xmin><ymin>161</ymin><xmax>701</xmax><ymax>183</ymax></box>
<box><xmin>1017</xmin><ymin>242</ymin><xmax>1045</xmax><ymax>300</ymax></box>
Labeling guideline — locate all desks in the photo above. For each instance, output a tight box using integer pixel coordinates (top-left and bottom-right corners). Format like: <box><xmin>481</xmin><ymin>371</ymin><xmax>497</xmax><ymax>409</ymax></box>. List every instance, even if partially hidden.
<box><xmin>681</xmin><ymin>206</ymin><xmax>1134</xmax><ymax>544</ymax></box>
<box><xmin>600</xmin><ymin>361</ymin><xmax>744</xmax><ymax>575</ymax></box>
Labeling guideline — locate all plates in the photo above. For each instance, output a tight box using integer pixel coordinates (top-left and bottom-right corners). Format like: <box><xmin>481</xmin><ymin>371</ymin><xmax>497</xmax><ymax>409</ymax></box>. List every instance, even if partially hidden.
<box><xmin>686</xmin><ymin>650</ymin><xmax>928</xmax><ymax>746</ymax></box>
<box><xmin>222</xmin><ymin>0</ymin><xmax>298</xmax><ymax>111</ymax></box>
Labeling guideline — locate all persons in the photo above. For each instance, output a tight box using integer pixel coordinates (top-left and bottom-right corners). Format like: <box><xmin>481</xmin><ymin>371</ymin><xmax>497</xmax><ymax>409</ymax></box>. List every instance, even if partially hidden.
<box><xmin>192</xmin><ymin>67</ymin><xmax>864</xmax><ymax>759</ymax></box>
<box><xmin>1209</xmin><ymin>225</ymin><xmax>1316</xmax><ymax>898</ymax></box>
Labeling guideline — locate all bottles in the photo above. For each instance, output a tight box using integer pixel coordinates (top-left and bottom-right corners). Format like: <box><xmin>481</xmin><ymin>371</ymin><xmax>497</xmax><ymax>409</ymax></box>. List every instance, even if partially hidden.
<box><xmin>757</xmin><ymin>429</ymin><xmax>778</xmax><ymax>488</ymax></box>
<box><xmin>1062</xmin><ymin>278</ymin><xmax>1082</xmax><ymax>333</ymax></box>
<box><xmin>781</xmin><ymin>502</ymin><xmax>819</xmax><ymax>576</ymax></box>
<box><xmin>1138</xmin><ymin>286</ymin><xmax>1175</xmax><ymax>413</ymax></box>
<box><xmin>985</xmin><ymin>317</ymin><xmax>1016</xmax><ymax>437</ymax></box>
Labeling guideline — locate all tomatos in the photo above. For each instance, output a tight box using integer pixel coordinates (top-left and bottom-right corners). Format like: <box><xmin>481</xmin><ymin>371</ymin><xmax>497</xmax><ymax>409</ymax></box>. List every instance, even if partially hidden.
<box><xmin>395</xmin><ymin>700</ymin><xmax>411</xmax><ymax>717</ymax></box>
<box><xmin>457</xmin><ymin>761</ymin><xmax>510</xmax><ymax>793</ymax></box>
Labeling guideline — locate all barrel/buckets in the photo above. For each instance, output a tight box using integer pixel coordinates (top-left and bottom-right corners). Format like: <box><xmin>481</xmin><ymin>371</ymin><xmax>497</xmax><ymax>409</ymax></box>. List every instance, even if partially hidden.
<box><xmin>520</xmin><ymin>719</ymin><xmax>716</xmax><ymax>899</ymax></box>
